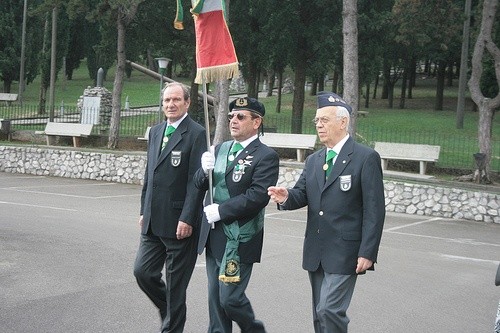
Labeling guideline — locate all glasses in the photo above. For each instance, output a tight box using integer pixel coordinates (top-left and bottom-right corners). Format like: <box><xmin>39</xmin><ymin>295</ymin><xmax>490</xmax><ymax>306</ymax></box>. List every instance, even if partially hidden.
<box><xmin>228</xmin><ymin>113</ymin><xmax>259</xmax><ymax>120</ymax></box>
<box><xmin>311</xmin><ymin>116</ymin><xmax>343</xmax><ymax>125</ymax></box>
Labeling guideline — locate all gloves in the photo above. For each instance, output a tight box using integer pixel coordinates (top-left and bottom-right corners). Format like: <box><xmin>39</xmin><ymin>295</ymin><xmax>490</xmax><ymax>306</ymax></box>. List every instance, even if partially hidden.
<box><xmin>204</xmin><ymin>203</ymin><xmax>222</xmax><ymax>223</ymax></box>
<box><xmin>203</xmin><ymin>145</ymin><xmax>216</xmax><ymax>175</ymax></box>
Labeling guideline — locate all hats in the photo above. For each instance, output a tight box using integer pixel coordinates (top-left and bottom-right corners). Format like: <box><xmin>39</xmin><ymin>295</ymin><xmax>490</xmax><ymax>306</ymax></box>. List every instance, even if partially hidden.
<box><xmin>229</xmin><ymin>96</ymin><xmax>265</xmax><ymax>116</ymax></box>
<box><xmin>317</xmin><ymin>91</ymin><xmax>352</xmax><ymax>114</ymax></box>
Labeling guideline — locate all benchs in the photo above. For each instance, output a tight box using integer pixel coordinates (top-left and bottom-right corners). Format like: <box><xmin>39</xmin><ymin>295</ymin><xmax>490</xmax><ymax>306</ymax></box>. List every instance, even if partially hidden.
<box><xmin>375</xmin><ymin>142</ymin><xmax>441</xmax><ymax>175</ymax></box>
<box><xmin>259</xmin><ymin>132</ymin><xmax>317</xmax><ymax>162</ymax></box>
<box><xmin>34</xmin><ymin>122</ymin><xmax>93</xmax><ymax>147</ymax></box>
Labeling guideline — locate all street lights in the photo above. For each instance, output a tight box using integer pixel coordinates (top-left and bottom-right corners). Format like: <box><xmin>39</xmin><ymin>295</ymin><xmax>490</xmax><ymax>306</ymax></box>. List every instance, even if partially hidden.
<box><xmin>156</xmin><ymin>56</ymin><xmax>173</xmax><ymax>123</ymax></box>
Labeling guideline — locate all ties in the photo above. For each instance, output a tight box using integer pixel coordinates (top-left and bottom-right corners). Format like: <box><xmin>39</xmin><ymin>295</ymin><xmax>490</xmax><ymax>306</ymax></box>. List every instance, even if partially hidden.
<box><xmin>161</xmin><ymin>125</ymin><xmax>177</xmax><ymax>151</ymax></box>
<box><xmin>325</xmin><ymin>150</ymin><xmax>337</xmax><ymax>177</ymax></box>
<box><xmin>227</xmin><ymin>143</ymin><xmax>244</xmax><ymax>168</ymax></box>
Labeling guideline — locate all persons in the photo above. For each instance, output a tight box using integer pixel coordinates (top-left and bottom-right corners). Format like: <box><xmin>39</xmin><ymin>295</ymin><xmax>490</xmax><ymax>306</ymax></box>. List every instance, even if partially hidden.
<box><xmin>133</xmin><ymin>82</ymin><xmax>208</xmax><ymax>333</ymax></box>
<box><xmin>194</xmin><ymin>97</ymin><xmax>279</xmax><ymax>333</ymax></box>
<box><xmin>267</xmin><ymin>91</ymin><xmax>386</xmax><ymax>333</ymax></box>
